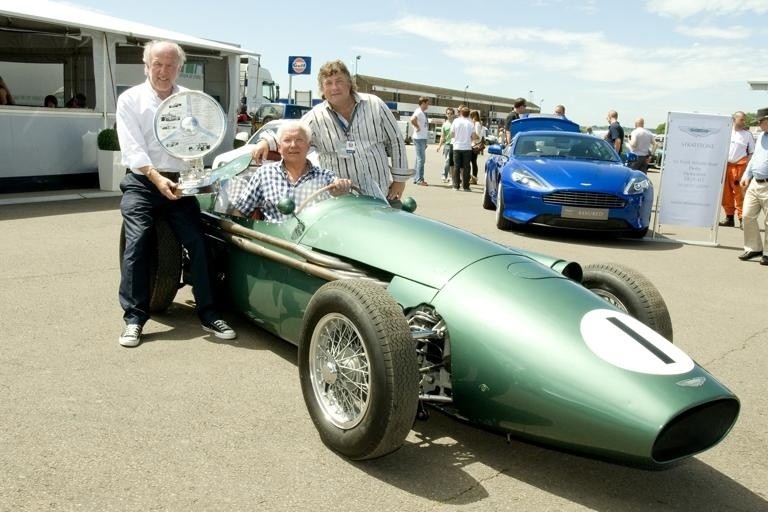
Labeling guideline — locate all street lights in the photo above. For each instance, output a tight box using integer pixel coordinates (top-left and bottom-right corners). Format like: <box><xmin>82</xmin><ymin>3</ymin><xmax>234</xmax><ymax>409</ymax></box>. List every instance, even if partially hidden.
<box><xmin>353</xmin><ymin>52</ymin><xmax>361</xmax><ymax>97</ymax></box>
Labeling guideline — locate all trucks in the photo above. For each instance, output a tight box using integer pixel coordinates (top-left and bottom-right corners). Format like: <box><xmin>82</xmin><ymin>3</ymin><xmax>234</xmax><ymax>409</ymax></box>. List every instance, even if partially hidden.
<box><xmin>0</xmin><ymin>35</ymin><xmax>279</xmax><ymax>122</ymax></box>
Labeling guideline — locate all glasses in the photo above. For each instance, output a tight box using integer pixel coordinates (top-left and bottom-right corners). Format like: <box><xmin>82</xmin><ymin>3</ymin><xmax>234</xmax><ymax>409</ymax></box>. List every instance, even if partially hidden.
<box><xmin>522</xmin><ymin>104</ymin><xmax>525</xmax><ymax>107</ymax></box>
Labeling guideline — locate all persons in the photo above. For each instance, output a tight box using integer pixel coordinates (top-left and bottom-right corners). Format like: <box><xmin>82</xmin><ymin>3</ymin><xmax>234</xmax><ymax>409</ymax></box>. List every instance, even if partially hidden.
<box><xmin>410</xmin><ymin>94</ymin><xmax>430</xmax><ymax>189</ymax></box>
<box><xmin>604</xmin><ymin>111</ymin><xmax>625</xmax><ymax>157</ymax></box>
<box><xmin>239</xmin><ymin>96</ymin><xmax>250</xmax><ymax>124</ymax></box>
<box><xmin>505</xmin><ymin>97</ymin><xmax>526</xmax><ymax>146</ymax></box>
<box><xmin>64</xmin><ymin>92</ymin><xmax>87</xmax><ymax>108</ymax></box>
<box><xmin>435</xmin><ymin>107</ymin><xmax>456</xmax><ymax>183</ymax></box>
<box><xmin>251</xmin><ymin>58</ymin><xmax>417</xmax><ymax>203</ymax></box>
<box><xmin>230</xmin><ymin>120</ymin><xmax>354</xmax><ymax>221</ymax></box>
<box><xmin>450</xmin><ymin>107</ymin><xmax>476</xmax><ymax>193</ymax></box>
<box><xmin>554</xmin><ymin>105</ymin><xmax>565</xmax><ymax>116</ymax></box>
<box><xmin>44</xmin><ymin>95</ymin><xmax>58</xmax><ymax>108</ymax></box>
<box><xmin>627</xmin><ymin>117</ymin><xmax>658</xmax><ymax>175</ymax></box>
<box><xmin>469</xmin><ymin>111</ymin><xmax>482</xmax><ymax>184</ymax></box>
<box><xmin>457</xmin><ymin>105</ymin><xmax>465</xmax><ymax>117</ymax></box>
<box><xmin>478</xmin><ymin>124</ymin><xmax>488</xmax><ymax>156</ymax></box>
<box><xmin>736</xmin><ymin>107</ymin><xmax>768</xmax><ymax>266</ymax></box>
<box><xmin>0</xmin><ymin>75</ymin><xmax>16</xmax><ymax>105</ymax></box>
<box><xmin>719</xmin><ymin>110</ymin><xmax>756</xmax><ymax>231</ymax></box>
<box><xmin>115</xmin><ymin>37</ymin><xmax>239</xmax><ymax>349</ymax></box>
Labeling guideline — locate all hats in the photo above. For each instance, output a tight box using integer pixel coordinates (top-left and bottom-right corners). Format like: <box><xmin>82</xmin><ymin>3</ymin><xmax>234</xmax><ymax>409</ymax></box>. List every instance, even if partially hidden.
<box><xmin>749</xmin><ymin>107</ymin><xmax>767</xmax><ymax>124</ymax></box>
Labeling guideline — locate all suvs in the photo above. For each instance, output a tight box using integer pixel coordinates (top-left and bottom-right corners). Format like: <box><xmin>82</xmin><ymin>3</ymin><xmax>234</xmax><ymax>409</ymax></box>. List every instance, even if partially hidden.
<box><xmin>252</xmin><ymin>102</ymin><xmax>313</xmax><ymax>137</ymax></box>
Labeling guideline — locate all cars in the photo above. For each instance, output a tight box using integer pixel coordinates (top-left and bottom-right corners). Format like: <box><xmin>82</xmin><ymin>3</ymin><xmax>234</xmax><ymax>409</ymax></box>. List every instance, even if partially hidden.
<box><xmin>483</xmin><ymin>128</ymin><xmax>666</xmax><ymax>172</ymax></box>
<box><xmin>481</xmin><ymin>115</ymin><xmax>654</xmax><ymax>241</ymax></box>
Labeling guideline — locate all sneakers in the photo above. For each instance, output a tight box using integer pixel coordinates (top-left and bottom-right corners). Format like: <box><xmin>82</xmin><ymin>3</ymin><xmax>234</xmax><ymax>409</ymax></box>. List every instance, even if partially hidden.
<box><xmin>118</xmin><ymin>324</ymin><xmax>142</xmax><ymax>347</ymax></box>
<box><xmin>414</xmin><ymin>175</ymin><xmax>476</xmax><ymax>191</ymax></box>
<box><xmin>199</xmin><ymin>319</ymin><xmax>236</xmax><ymax>340</ymax></box>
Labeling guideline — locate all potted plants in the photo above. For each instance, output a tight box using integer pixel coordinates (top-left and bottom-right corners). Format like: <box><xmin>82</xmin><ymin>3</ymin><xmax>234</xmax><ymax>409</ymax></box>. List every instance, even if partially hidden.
<box><xmin>96</xmin><ymin>127</ymin><xmax>126</xmax><ymax>192</ymax></box>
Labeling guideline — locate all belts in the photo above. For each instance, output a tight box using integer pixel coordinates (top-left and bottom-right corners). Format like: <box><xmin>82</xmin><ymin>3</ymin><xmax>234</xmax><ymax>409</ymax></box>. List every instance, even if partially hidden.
<box><xmin>755</xmin><ymin>178</ymin><xmax>767</xmax><ymax>182</ymax></box>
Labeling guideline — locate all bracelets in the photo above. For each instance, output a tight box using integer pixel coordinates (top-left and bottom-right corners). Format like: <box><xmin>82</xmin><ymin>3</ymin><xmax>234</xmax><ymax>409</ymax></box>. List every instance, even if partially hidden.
<box><xmin>650</xmin><ymin>155</ymin><xmax>654</xmax><ymax>157</ymax></box>
<box><xmin>146</xmin><ymin>167</ymin><xmax>157</xmax><ymax>181</ymax></box>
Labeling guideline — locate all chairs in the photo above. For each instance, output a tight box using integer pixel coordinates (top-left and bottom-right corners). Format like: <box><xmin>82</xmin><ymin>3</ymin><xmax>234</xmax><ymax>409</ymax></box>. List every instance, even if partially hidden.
<box><xmin>522</xmin><ymin>141</ymin><xmax>593</xmax><ymax>157</ymax></box>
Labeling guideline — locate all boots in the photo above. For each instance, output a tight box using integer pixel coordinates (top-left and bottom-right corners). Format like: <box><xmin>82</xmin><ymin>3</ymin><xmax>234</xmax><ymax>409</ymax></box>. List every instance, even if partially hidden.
<box><xmin>719</xmin><ymin>215</ymin><xmax>733</xmax><ymax>226</ymax></box>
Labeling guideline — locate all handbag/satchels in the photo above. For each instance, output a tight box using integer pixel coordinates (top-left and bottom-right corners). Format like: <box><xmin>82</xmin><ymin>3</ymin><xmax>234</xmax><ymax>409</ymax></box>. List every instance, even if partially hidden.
<box><xmin>470</xmin><ymin>141</ymin><xmax>485</xmax><ymax>154</ymax></box>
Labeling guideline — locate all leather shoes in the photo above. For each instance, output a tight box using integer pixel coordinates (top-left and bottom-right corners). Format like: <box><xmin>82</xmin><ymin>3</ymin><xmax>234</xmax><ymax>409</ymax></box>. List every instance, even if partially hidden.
<box><xmin>738</xmin><ymin>251</ymin><xmax>762</xmax><ymax>259</ymax></box>
<box><xmin>759</xmin><ymin>256</ymin><xmax>767</xmax><ymax>265</ymax></box>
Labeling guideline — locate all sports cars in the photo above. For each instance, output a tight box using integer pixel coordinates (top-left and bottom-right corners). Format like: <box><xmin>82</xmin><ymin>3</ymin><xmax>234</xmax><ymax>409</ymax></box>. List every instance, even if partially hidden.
<box><xmin>117</xmin><ymin>164</ymin><xmax>742</xmax><ymax>471</ymax></box>
